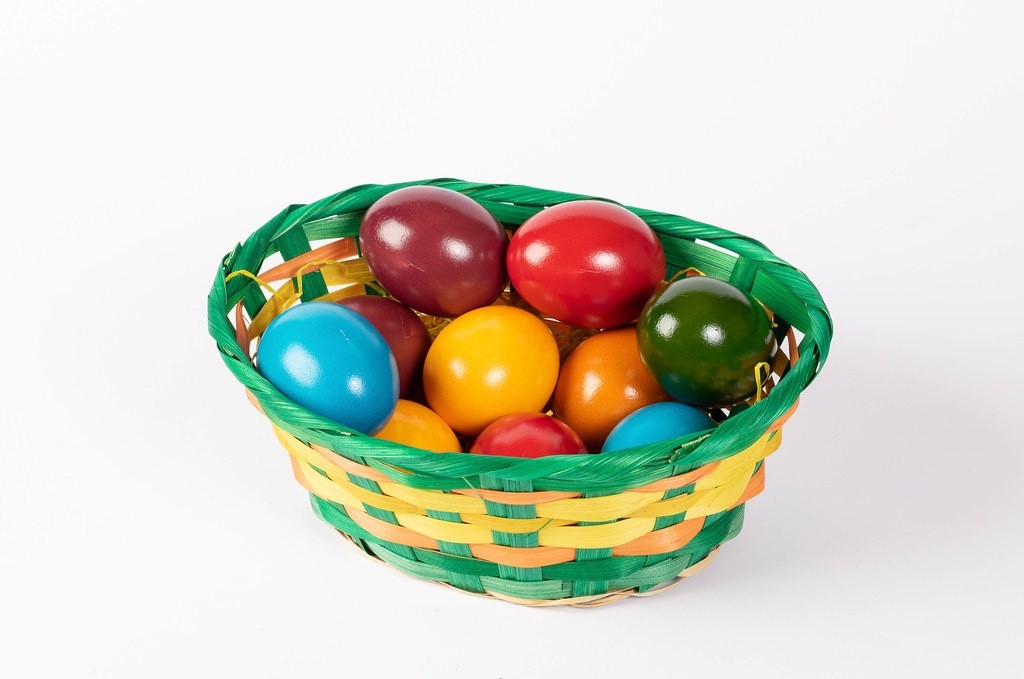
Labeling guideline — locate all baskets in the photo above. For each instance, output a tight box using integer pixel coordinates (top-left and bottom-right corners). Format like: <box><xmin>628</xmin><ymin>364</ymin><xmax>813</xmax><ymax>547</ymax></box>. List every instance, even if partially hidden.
<box><xmin>208</xmin><ymin>178</ymin><xmax>833</xmax><ymax>609</ymax></box>
